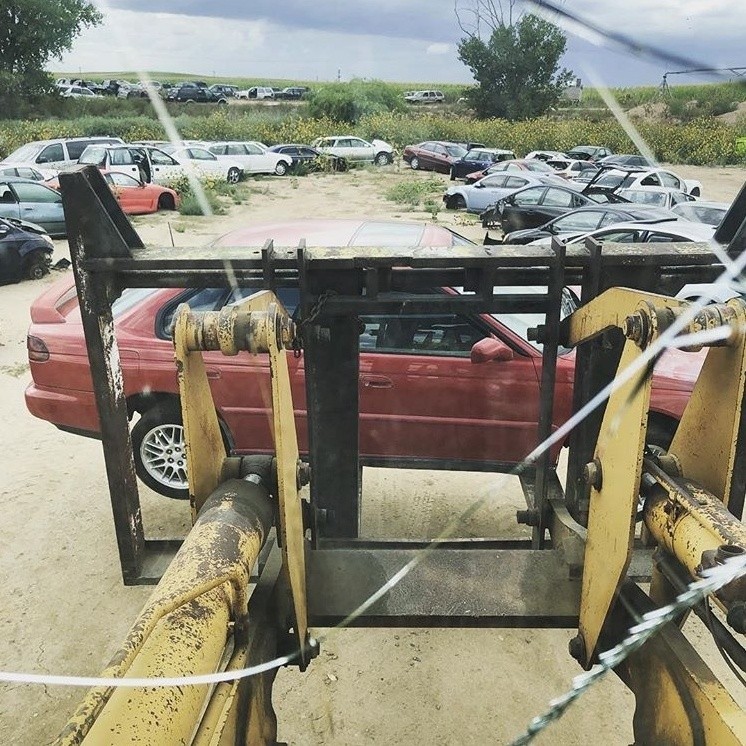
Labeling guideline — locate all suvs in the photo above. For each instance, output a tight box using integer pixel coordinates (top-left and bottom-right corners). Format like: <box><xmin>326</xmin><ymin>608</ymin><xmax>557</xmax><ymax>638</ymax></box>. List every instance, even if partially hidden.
<box><xmin>311</xmin><ymin>136</ymin><xmax>393</xmax><ymax>167</ymax></box>
<box><xmin>77</xmin><ymin>143</ymin><xmax>185</xmax><ymax>186</ymax></box>
<box><xmin>404</xmin><ymin>90</ymin><xmax>446</xmax><ymax>105</ymax></box>
<box><xmin>0</xmin><ymin>136</ymin><xmax>125</xmax><ymax>172</ymax></box>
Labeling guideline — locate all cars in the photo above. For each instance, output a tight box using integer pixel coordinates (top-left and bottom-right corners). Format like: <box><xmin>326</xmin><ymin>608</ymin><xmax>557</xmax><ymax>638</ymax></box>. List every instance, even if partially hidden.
<box><xmin>155</xmin><ymin>146</ymin><xmax>244</xmax><ymax>184</ymax></box>
<box><xmin>443</xmin><ymin>172</ymin><xmax>582</xmax><ymax>215</ymax></box>
<box><xmin>502</xmin><ymin>203</ymin><xmax>730</xmax><ymax>292</ymax></box>
<box><xmin>54</xmin><ymin>77</ymin><xmax>309</xmax><ymax>105</ymax></box>
<box><xmin>464</xmin><ymin>151</ymin><xmax>648</xmax><ymax>183</ymax></box>
<box><xmin>206</xmin><ymin>143</ymin><xmax>292</xmax><ymax>176</ymax></box>
<box><xmin>45</xmin><ymin>170</ymin><xmax>179</xmax><ymax>215</ymax></box>
<box><xmin>596</xmin><ymin>168</ymin><xmax>702</xmax><ymax>202</ymax></box>
<box><xmin>566</xmin><ymin>146</ymin><xmax>612</xmax><ymax>163</ymax></box>
<box><xmin>268</xmin><ymin>144</ymin><xmax>347</xmax><ymax>174</ymax></box>
<box><xmin>403</xmin><ymin>141</ymin><xmax>468</xmax><ymax>175</ymax></box>
<box><xmin>0</xmin><ymin>217</ymin><xmax>54</xmax><ymax>286</ymax></box>
<box><xmin>25</xmin><ymin>219</ymin><xmax>711</xmax><ymax>501</ymax></box>
<box><xmin>495</xmin><ymin>163</ymin><xmax>644</xmax><ymax>232</ymax></box>
<box><xmin>0</xmin><ymin>163</ymin><xmax>67</xmax><ymax>237</ymax></box>
<box><xmin>449</xmin><ymin>148</ymin><xmax>517</xmax><ymax>181</ymax></box>
